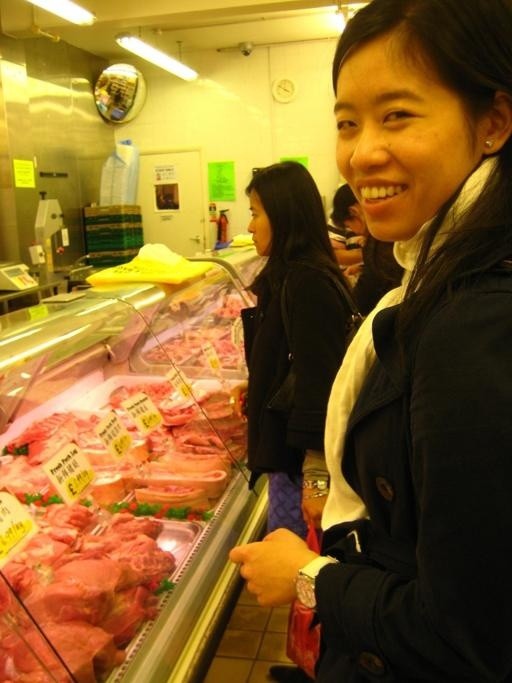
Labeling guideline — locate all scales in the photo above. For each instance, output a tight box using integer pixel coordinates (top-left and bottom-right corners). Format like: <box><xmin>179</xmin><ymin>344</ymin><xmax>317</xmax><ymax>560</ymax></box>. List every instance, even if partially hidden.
<box><xmin>0</xmin><ymin>261</ymin><xmax>40</xmax><ymax>290</ymax></box>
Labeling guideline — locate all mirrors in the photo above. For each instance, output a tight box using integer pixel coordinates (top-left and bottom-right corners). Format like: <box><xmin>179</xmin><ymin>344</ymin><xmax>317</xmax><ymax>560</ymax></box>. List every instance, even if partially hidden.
<box><xmin>94</xmin><ymin>63</ymin><xmax>146</xmax><ymax>123</ymax></box>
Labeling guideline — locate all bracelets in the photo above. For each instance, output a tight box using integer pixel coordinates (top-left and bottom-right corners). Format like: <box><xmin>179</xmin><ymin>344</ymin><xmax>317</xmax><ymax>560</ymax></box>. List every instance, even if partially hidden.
<box><xmin>303</xmin><ymin>491</ymin><xmax>328</xmax><ymax>499</ymax></box>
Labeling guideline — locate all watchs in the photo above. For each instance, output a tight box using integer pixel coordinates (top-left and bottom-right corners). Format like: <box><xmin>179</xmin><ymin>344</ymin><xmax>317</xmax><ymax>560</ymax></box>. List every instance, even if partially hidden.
<box><xmin>301</xmin><ymin>478</ymin><xmax>328</xmax><ymax>491</ymax></box>
<box><xmin>293</xmin><ymin>553</ymin><xmax>339</xmax><ymax>614</ymax></box>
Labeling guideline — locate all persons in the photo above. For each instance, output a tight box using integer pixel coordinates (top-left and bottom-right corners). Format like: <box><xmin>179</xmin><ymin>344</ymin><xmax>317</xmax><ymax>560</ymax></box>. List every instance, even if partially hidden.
<box><xmin>99</xmin><ymin>76</ymin><xmax>109</xmax><ymax>97</ymax></box>
<box><xmin>229</xmin><ymin>0</ymin><xmax>511</xmax><ymax>683</ymax></box>
<box><xmin>327</xmin><ymin>185</ymin><xmax>405</xmax><ymax>317</ymax></box>
<box><xmin>107</xmin><ymin>82</ymin><xmax>113</xmax><ymax>94</ymax></box>
<box><xmin>238</xmin><ymin>157</ymin><xmax>367</xmax><ymax>682</ymax></box>
<box><xmin>114</xmin><ymin>88</ymin><xmax>123</xmax><ymax>106</ymax></box>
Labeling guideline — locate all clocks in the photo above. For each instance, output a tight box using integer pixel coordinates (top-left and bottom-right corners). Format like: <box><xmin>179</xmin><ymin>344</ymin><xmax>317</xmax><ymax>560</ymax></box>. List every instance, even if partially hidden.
<box><xmin>272</xmin><ymin>78</ymin><xmax>296</xmax><ymax>104</ymax></box>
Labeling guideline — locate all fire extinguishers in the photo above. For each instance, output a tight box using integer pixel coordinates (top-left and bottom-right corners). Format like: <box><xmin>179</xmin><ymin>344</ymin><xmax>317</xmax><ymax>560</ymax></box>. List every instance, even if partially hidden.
<box><xmin>218</xmin><ymin>208</ymin><xmax>228</xmax><ymax>242</ymax></box>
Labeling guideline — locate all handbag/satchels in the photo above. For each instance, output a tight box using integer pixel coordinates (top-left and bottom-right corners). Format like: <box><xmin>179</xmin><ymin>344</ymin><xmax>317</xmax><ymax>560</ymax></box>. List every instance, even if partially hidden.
<box><xmin>286</xmin><ymin>517</ymin><xmax>329</xmax><ymax>678</ymax></box>
<box><xmin>266</xmin><ymin>261</ymin><xmax>366</xmax><ymax>423</ymax></box>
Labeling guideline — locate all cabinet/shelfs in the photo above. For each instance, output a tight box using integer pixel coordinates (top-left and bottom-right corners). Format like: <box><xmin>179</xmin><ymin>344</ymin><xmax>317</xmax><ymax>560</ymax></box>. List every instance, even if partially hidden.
<box><xmin>2</xmin><ymin>248</ymin><xmax>269</xmax><ymax>682</ymax></box>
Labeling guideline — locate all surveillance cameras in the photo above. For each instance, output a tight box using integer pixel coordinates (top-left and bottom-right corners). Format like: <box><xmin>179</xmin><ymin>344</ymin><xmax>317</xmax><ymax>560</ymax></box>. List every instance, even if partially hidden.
<box><xmin>238</xmin><ymin>42</ymin><xmax>254</xmax><ymax>57</ymax></box>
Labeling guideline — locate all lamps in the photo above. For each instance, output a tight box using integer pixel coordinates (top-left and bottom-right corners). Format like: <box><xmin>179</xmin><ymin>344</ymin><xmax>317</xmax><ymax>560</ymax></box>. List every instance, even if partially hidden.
<box><xmin>114</xmin><ymin>27</ymin><xmax>198</xmax><ymax>82</ymax></box>
<box><xmin>29</xmin><ymin>0</ymin><xmax>96</xmax><ymax>27</ymax></box>
